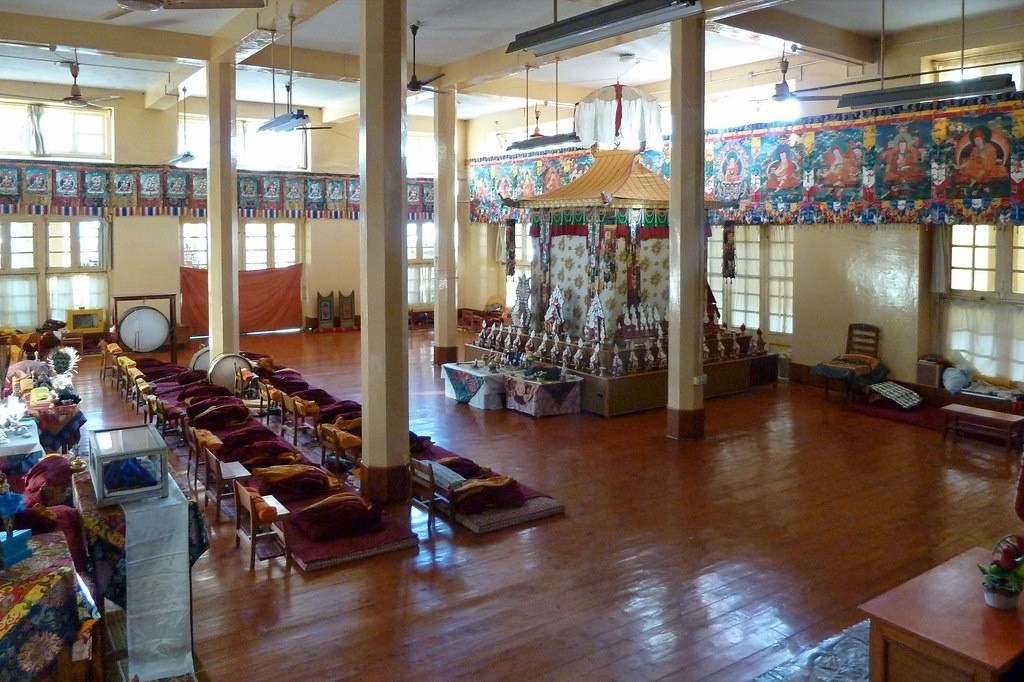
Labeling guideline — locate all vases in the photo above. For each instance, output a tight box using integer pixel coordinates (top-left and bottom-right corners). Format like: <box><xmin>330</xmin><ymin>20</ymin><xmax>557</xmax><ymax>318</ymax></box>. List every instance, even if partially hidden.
<box><xmin>983</xmin><ymin>587</ymin><xmax>1017</xmax><ymax>609</ymax></box>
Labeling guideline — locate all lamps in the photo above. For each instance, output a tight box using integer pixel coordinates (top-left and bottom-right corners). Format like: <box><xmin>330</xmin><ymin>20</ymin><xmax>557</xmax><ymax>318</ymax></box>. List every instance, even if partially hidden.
<box><xmin>258</xmin><ymin>16</ymin><xmax>311</xmax><ymax>133</ymax></box>
<box><xmin>838</xmin><ymin>0</ymin><xmax>1015</xmax><ymax>110</ymax></box>
<box><xmin>504</xmin><ymin>0</ymin><xmax>705</xmax><ymax>56</ymax></box>
<box><xmin>168</xmin><ymin>88</ymin><xmax>196</xmax><ymax>164</ymax></box>
<box><xmin>506</xmin><ymin>57</ymin><xmax>582</xmax><ymax>151</ymax></box>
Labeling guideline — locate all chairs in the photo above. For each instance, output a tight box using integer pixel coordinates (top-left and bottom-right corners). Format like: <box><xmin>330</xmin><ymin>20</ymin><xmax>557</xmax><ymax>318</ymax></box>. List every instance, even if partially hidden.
<box><xmin>0</xmin><ymin>331</ymin><xmax>456</xmax><ymax>574</ymax></box>
<box><xmin>463</xmin><ymin>310</ymin><xmax>481</xmax><ymax>333</ymax></box>
<box><xmin>810</xmin><ymin>324</ymin><xmax>879</xmax><ymax>412</ymax></box>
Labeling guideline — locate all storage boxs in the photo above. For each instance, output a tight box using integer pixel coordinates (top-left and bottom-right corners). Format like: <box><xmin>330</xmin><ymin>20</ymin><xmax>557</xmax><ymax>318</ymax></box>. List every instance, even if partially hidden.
<box><xmin>917</xmin><ymin>359</ymin><xmax>950</xmax><ymax>388</ymax></box>
<box><xmin>88</xmin><ymin>423</ymin><xmax>169</xmax><ymax>509</ymax></box>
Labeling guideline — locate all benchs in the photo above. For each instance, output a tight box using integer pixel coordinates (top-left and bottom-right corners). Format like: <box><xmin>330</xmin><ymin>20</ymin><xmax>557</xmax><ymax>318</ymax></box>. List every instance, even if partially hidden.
<box><xmin>940</xmin><ymin>404</ymin><xmax>1024</xmax><ymax>457</ymax></box>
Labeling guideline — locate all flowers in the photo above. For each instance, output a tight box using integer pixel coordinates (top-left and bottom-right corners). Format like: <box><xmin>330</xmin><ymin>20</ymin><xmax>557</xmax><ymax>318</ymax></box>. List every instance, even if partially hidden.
<box><xmin>975</xmin><ymin>534</ymin><xmax>1024</xmax><ymax>594</ymax></box>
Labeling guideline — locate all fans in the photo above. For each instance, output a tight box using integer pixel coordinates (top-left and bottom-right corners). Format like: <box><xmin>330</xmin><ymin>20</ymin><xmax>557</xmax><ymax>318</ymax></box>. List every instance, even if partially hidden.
<box><xmin>406</xmin><ymin>25</ymin><xmax>451</xmax><ymax>95</ymax></box>
<box><xmin>529</xmin><ymin>103</ymin><xmax>545</xmax><ymax>139</ymax></box>
<box><xmin>96</xmin><ymin>0</ymin><xmax>268</xmax><ymax>22</ymax></box>
<box><xmin>45</xmin><ymin>47</ymin><xmax>123</xmax><ymax>108</ymax></box>
<box><xmin>749</xmin><ymin>37</ymin><xmax>841</xmax><ymax>103</ymax></box>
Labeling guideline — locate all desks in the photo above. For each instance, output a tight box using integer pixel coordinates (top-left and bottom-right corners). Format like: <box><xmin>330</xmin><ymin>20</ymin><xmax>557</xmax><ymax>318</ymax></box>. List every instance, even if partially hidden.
<box><xmin>503</xmin><ymin>371</ymin><xmax>584</xmax><ymax>420</ymax></box>
<box><xmin>0</xmin><ymin>396</ymin><xmax>211</xmax><ymax>682</ymax></box>
<box><xmin>440</xmin><ymin>360</ymin><xmax>524</xmax><ymax>411</ymax></box>
<box><xmin>856</xmin><ymin>547</ymin><xmax>1024</xmax><ymax>682</ymax></box>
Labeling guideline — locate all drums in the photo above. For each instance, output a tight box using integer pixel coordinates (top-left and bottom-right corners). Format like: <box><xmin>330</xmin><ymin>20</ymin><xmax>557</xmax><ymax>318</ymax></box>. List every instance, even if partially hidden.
<box><xmin>206</xmin><ymin>353</ymin><xmax>257</xmax><ymax>394</ymax></box>
<box><xmin>187</xmin><ymin>346</ymin><xmax>213</xmax><ymax>374</ymax></box>
<box><xmin>116</xmin><ymin>305</ymin><xmax>171</xmax><ymax>354</ymax></box>
